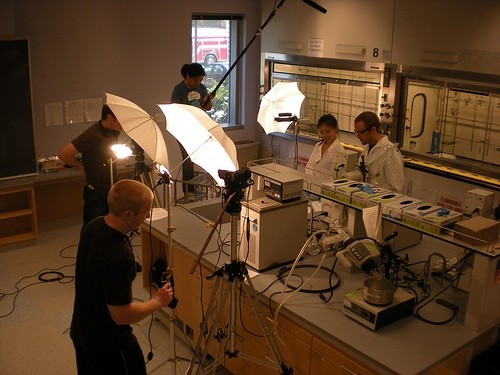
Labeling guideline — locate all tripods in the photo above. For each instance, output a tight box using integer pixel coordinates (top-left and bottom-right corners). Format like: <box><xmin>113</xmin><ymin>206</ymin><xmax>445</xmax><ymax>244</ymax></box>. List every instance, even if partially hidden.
<box><xmin>184</xmin><ymin>214</ymin><xmax>293</xmax><ymax>375</ymax></box>
<box><xmin>147</xmin><ymin>182</ymin><xmax>204</xmax><ymax>375</ymax></box>
<box><xmin>133</xmin><ymin>164</ymin><xmax>163</xmax><ymax>209</ymax></box>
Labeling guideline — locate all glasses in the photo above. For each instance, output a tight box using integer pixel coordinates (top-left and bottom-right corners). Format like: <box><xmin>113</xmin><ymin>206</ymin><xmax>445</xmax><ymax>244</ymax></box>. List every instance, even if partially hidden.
<box><xmin>354</xmin><ymin>129</ymin><xmax>370</xmax><ymax>134</ymax></box>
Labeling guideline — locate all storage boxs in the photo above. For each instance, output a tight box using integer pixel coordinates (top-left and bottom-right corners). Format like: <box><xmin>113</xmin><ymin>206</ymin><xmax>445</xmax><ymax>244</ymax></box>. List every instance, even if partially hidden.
<box><xmin>232</xmin><ymin>140</ymin><xmax>259</xmax><ymax>170</ymax></box>
<box><xmin>452</xmin><ymin>216</ymin><xmax>499</xmax><ymax>246</ymax></box>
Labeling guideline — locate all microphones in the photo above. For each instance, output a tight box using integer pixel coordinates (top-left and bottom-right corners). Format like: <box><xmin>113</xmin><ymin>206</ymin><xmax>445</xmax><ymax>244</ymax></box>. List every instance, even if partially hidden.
<box><xmin>302</xmin><ymin>0</ymin><xmax>327</xmax><ymax>14</ymax></box>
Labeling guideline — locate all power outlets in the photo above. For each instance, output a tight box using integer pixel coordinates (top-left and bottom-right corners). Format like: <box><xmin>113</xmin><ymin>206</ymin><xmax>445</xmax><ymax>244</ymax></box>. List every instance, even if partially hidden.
<box><xmin>308</xmin><ymin>38</ymin><xmax>323</xmax><ymax>56</ymax></box>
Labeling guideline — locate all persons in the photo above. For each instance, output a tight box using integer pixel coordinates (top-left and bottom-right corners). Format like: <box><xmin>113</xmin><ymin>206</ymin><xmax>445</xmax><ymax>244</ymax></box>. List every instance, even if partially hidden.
<box><xmin>170</xmin><ymin>63</ymin><xmax>216</xmax><ymax>194</ymax></box>
<box><xmin>58</xmin><ymin>103</ymin><xmax>122</xmax><ymax>225</ymax></box>
<box><xmin>354</xmin><ymin>111</ymin><xmax>405</xmax><ymax>194</ymax></box>
<box><xmin>305</xmin><ymin>114</ymin><xmax>345</xmax><ymax>178</ymax></box>
<box><xmin>69</xmin><ymin>179</ymin><xmax>173</xmax><ymax>375</ymax></box>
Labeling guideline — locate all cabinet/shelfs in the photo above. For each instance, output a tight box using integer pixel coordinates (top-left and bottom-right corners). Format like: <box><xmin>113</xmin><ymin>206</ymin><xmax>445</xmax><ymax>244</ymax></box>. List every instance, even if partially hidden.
<box><xmin>0</xmin><ymin>167</ymin><xmax>81</xmax><ymax>246</ymax></box>
<box><xmin>142</xmin><ymin>51</ymin><xmax>500</xmax><ymax>375</ymax></box>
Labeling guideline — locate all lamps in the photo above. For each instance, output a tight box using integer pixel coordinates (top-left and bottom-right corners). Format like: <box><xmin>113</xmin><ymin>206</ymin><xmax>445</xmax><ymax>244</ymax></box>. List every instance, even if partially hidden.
<box><xmin>110</xmin><ymin>144</ymin><xmax>133</xmax><ymax>159</ymax></box>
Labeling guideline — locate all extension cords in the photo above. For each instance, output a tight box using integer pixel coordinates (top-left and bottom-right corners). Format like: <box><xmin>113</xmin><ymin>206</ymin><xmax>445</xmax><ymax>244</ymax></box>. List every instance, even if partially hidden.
<box><xmin>318</xmin><ymin>234</ymin><xmax>344</xmax><ymax>246</ymax></box>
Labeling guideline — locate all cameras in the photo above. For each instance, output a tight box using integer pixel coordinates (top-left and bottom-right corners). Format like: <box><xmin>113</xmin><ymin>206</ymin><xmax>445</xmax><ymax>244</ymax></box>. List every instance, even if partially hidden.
<box><xmin>218</xmin><ymin>167</ymin><xmax>254</xmax><ymax>214</ymax></box>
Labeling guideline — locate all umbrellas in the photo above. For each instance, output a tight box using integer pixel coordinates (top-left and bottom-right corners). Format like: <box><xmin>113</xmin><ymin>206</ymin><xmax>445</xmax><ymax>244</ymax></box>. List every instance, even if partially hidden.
<box><xmin>257</xmin><ymin>81</ymin><xmax>306</xmax><ymax>135</ymax></box>
<box><xmin>103</xmin><ymin>91</ymin><xmax>170</xmax><ymax>171</ymax></box>
<box><xmin>152</xmin><ymin>103</ymin><xmax>239</xmax><ymax>192</ymax></box>
